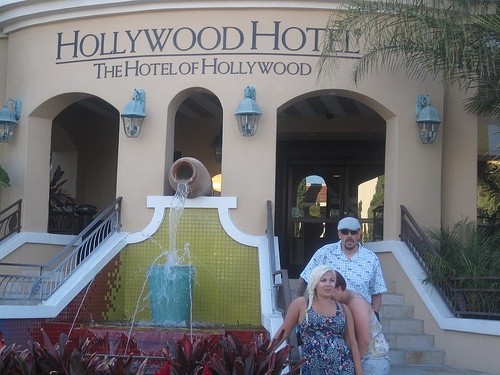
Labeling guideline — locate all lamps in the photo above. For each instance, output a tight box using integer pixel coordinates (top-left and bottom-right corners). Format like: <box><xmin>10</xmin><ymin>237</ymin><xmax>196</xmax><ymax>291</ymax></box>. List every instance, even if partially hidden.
<box><xmin>0</xmin><ymin>98</ymin><xmax>21</xmax><ymax>143</ymax></box>
<box><xmin>234</xmin><ymin>86</ymin><xmax>262</xmax><ymax>136</ymax></box>
<box><xmin>416</xmin><ymin>94</ymin><xmax>441</xmax><ymax>145</ymax></box>
<box><xmin>121</xmin><ymin>89</ymin><xmax>146</xmax><ymax>138</ymax></box>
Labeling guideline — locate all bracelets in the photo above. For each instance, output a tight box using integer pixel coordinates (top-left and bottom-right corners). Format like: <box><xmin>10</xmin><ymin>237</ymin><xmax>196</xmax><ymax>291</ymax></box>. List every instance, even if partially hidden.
<box><xmin>374</xmin><ymin>311</ymin><xmax>379</xmax><ymax>317</ymax></box>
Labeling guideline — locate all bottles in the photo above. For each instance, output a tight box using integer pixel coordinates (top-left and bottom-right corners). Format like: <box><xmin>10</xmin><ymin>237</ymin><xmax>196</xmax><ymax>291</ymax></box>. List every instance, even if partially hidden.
<box><xmin>168</xmin><ymin>157</ymin><xmax>212</xmax><ymax>197</ymax></box>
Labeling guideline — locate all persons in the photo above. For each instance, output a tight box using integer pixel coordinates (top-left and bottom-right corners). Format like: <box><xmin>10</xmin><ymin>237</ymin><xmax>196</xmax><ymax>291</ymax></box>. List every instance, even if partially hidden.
<box><xmin>295</xmin><ymin>217</ymin><xmax>387</xmax><ymax>318</ymax></box>
<box><xmin>330</xmin><ymin>270</ymin><xmax>390</xmax><ymax>375</ymax></box>
<box><xmin>267</xmin><ymin>265</ymin><xmax>361</xmax><ymax>375</ymax></box>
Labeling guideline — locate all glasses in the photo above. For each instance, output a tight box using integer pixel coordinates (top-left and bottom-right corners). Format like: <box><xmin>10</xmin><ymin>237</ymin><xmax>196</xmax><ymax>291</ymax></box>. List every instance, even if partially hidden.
<box><xmin>340</xmin><ymin>228</ymin><xmax>360</xmax><ymax>235</ymax></box>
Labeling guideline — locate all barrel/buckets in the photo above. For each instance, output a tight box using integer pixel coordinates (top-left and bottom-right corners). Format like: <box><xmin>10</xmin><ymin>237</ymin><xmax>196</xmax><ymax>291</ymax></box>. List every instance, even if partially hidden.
<box><xmin>148</xmin><ymin>264</ymin><xmax>194</xmax><ymax>326</ymax></box>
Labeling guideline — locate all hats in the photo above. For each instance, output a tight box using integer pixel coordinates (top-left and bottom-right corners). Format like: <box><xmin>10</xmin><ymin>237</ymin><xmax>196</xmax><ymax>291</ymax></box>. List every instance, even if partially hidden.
<box><xmin>337</xmin><ymin>216</ymin><xmax>361</xmax><ymax>231</ymax></box>
